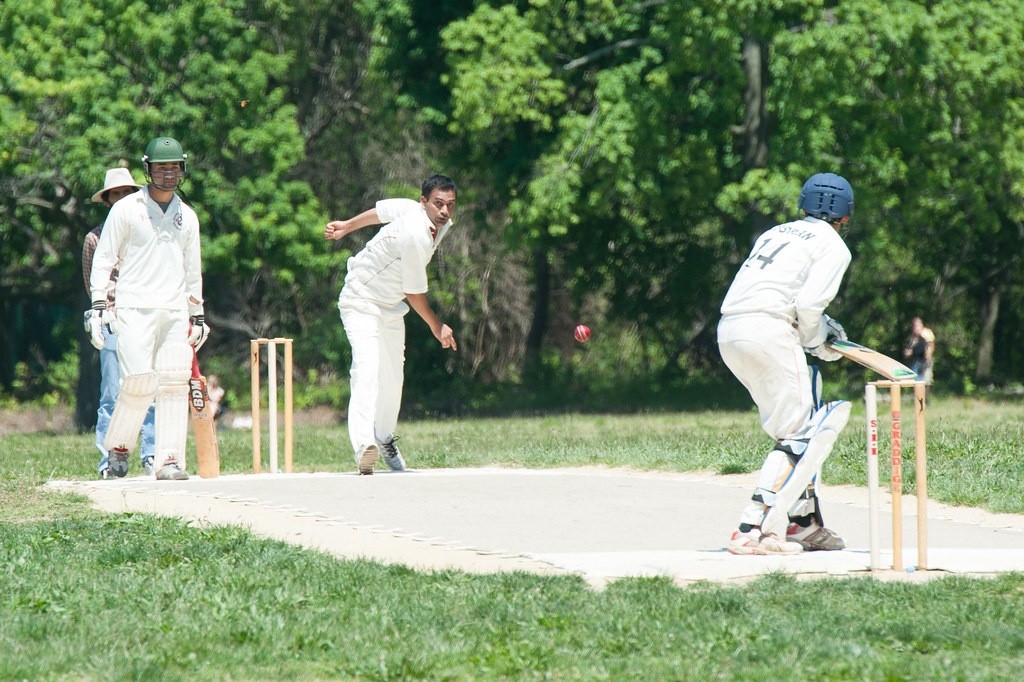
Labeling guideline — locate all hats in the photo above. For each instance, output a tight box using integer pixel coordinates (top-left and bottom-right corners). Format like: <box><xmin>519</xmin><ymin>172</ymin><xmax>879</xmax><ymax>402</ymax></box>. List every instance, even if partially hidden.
<box><xmin>91</xmin><ymin>168</ymin><xmax>145</xmax><ymax>203</ymax></box>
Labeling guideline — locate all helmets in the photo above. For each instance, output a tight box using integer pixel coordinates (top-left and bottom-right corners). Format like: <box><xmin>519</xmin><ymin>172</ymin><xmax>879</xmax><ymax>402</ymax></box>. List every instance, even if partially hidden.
<box><xmin>141</xmin><ymin>137</ymin><xmax>187</xmax><ymax>192</ymax></box>
<box><xmin>797</xmin><ymin>172</ymin><xmax>854</xmax><ymax>241</ymax></box>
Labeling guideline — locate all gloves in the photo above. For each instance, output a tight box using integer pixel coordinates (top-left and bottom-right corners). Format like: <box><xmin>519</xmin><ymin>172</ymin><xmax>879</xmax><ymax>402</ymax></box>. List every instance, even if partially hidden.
<box><xmin>823</xmin><ymin>314</ymin><xmax>848</xmax><ymax>342</ymax></box>
<box><xmin>188</xmin><ymin>315</ymin><xmax>204</xmax><ymax>346</ymax></box>
<box><xmin>84</xmin><ymin>300</ymin><xmax>119</xmax><ymax>350</ymax></box>
<box><xmin>804</xmin><ymin>343</ymin><xmax>843</xmax><ymax>362</ymax></box>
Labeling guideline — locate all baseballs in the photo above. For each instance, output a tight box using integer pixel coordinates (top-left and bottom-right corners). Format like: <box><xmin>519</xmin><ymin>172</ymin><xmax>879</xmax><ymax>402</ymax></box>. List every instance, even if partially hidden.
<box><xmin>574</xmin><ymin>324</ymin><xmax>592</xmax><ymax>342</ymax></box>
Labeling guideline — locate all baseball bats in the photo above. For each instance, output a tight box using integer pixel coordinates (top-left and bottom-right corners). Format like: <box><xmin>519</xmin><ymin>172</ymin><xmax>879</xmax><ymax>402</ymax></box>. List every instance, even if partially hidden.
<box><xmin>188</xmin><ymin>322</ymin><xmax>221</xmax><ymax>479</ymax></box>
<box><xmin>823</xmin><ymin>335</ymin><xmax>919</xmax><ymax>382</ymax></box>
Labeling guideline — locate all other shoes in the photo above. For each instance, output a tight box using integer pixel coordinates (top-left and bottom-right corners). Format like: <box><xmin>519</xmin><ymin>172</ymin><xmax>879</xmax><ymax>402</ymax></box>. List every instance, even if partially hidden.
<box><xmin>103</xmin><ymin>468</ymin><xmax>117</xmax><ymax>480</ymax></box>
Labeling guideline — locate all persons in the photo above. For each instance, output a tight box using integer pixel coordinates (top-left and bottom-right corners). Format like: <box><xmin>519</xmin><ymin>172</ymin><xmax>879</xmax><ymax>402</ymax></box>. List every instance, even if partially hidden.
<box><xmin>716</xmin><ymin>173</ymin><xmax>853</xmax><ymax>555</ymax></box>
<box><xmin>82</xmin><ymin>167</ymin><xmax>155</xmax><ymax>475</ymax></box>
<box><xmin>905</xmin><ymin>318</ymin><xmax>934</xmax><ymax>402</ymax></box>
<box><xmin>326</xmin><ymin>175</ymin><xmax>457</xmax><ymax>475</ymax></box>
<box><xmin>83</xmin><ymin>137</ymin><xmax>210</xmax><ymax>480</ymax></box>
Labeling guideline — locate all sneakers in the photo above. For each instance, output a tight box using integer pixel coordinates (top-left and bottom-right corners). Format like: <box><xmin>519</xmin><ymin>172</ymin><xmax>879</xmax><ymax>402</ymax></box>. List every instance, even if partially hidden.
<box><xmin>376</xmin><ymin>433</ymin><xmax>406</xmax><ymax>471</ymax></box>
<box><xmin>156</xmin><ymin>453</ymin><xmax>189</xmax><ymax>481</ymax></box>
<box><xmin>786</xmin><ymin>516</ymin><xmax>845</xmax><ymax>550</ymax></box>
<box><xmin>727</xmin><ymin>527</ymin><xmax>804</xmax><ymax>555</ymax></box>
<box><xmin>354</xmin><ymin>445</ymin><xmax>378</xmax><ymax>475</ymax></box>
<box><xmin>108</xmin><ymin>443</ymin><xmax>129</xmax><ymax>477</ymax></box>
